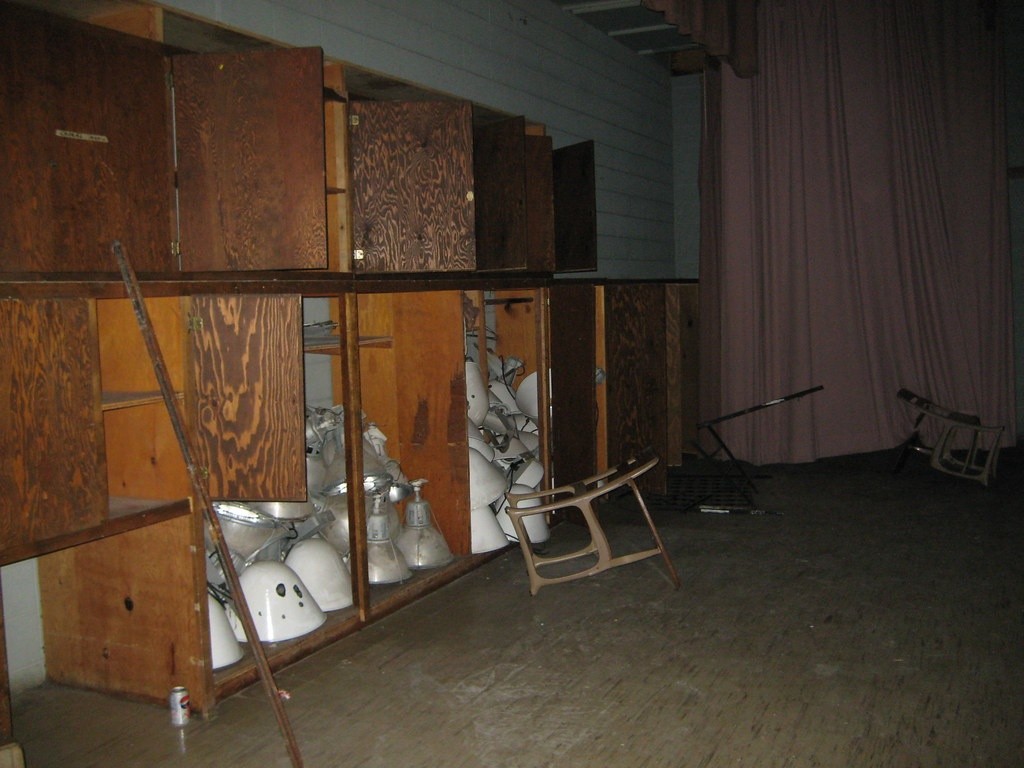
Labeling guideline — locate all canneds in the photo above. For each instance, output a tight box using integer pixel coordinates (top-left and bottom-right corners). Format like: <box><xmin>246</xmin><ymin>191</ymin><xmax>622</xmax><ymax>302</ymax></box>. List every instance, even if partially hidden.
<box><xmin>169</xmin><ymin>686</ymin><xmax>191</xmax><ymax>725</ymax></box>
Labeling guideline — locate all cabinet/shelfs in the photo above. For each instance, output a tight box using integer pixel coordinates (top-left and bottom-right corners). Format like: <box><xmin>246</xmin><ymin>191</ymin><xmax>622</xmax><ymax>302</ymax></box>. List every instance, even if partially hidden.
<box><xmin>0</xmin><ymin>0</ymin><xmax>700</xmax><ymax>768</ymax></box>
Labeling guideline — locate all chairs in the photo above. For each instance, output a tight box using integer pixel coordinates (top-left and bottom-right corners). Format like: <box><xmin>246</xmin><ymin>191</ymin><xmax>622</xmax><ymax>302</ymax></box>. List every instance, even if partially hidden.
<box><xmin>504</xmin><ymin>457</ymin><xmax>677</xmax><ymax>595</ymax></box>
<box><xmin>897</xmin><ymin>387</ymin><xmax>1005</xmax><ymax>486</ymax></box>
